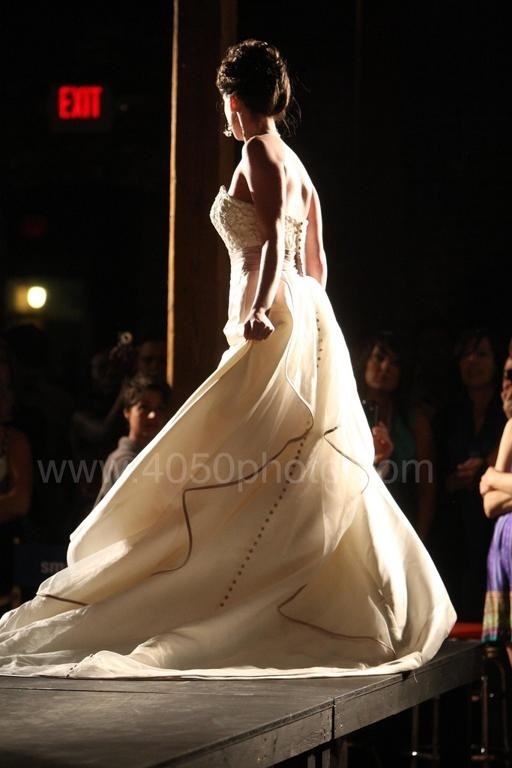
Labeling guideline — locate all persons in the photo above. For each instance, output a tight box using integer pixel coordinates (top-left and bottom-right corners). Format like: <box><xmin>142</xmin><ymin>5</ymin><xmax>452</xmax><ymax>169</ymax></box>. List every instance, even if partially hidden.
<box><xmin>3</xmin><ymin>339</ymin><xmax>510</xmax><ymax>660</ymax></box>
<box><xmin>206</xmin><ymin>38</ymin><xmax>378</xmax><ymax>672</ymax></box>
